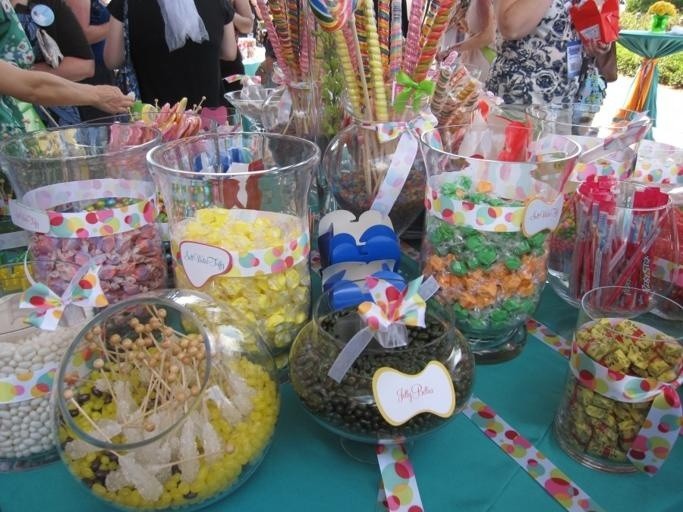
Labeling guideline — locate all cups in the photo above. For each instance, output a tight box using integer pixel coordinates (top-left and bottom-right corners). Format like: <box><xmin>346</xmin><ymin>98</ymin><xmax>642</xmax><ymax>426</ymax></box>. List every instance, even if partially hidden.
<box><xmin>552</xmin><ymin>279</ymin><xmax>683</xmax><ymax>478</ymax></box>
<box><xmin>1</xmin><ymin>119</ymin><xmax>171</xmax><ymax>300</ymax></box>
<box><xmin>570</xmin><ymin>178</ymin><xmax>679</xmax><ymax>312</ymax></box>
<box><xmin>144</xmin><ymin>128</ymin><xmax>323</xmax><ymax>374</ymax></box>
<box><xmin>522</xmin><ymin>101</ymin><xmax>653</xmax><ymax>295</ymax></box>
<box><xmin>414</xmin><ymin>121</ymin><xmax>583</xmax><ymax>356</ymax></box>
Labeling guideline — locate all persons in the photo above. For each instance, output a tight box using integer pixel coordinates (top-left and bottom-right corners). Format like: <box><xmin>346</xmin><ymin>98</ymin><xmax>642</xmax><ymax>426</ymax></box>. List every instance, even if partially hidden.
<box><xmin>0</xmin><ymin>0</ymin><xmax>496</xmax><ymax>217</ymax></box>
<box><xmin>486</xmin><ymin>1</ymin><xmax>620</xmax><ymax>133</ymax></box>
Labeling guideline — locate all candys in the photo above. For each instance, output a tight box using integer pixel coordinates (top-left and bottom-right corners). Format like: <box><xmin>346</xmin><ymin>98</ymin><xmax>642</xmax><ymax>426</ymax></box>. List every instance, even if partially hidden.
<box><xmin>0</xmin><ymin>1</ymin><xmax>683</xmax><ymax>510</ymax></box>
